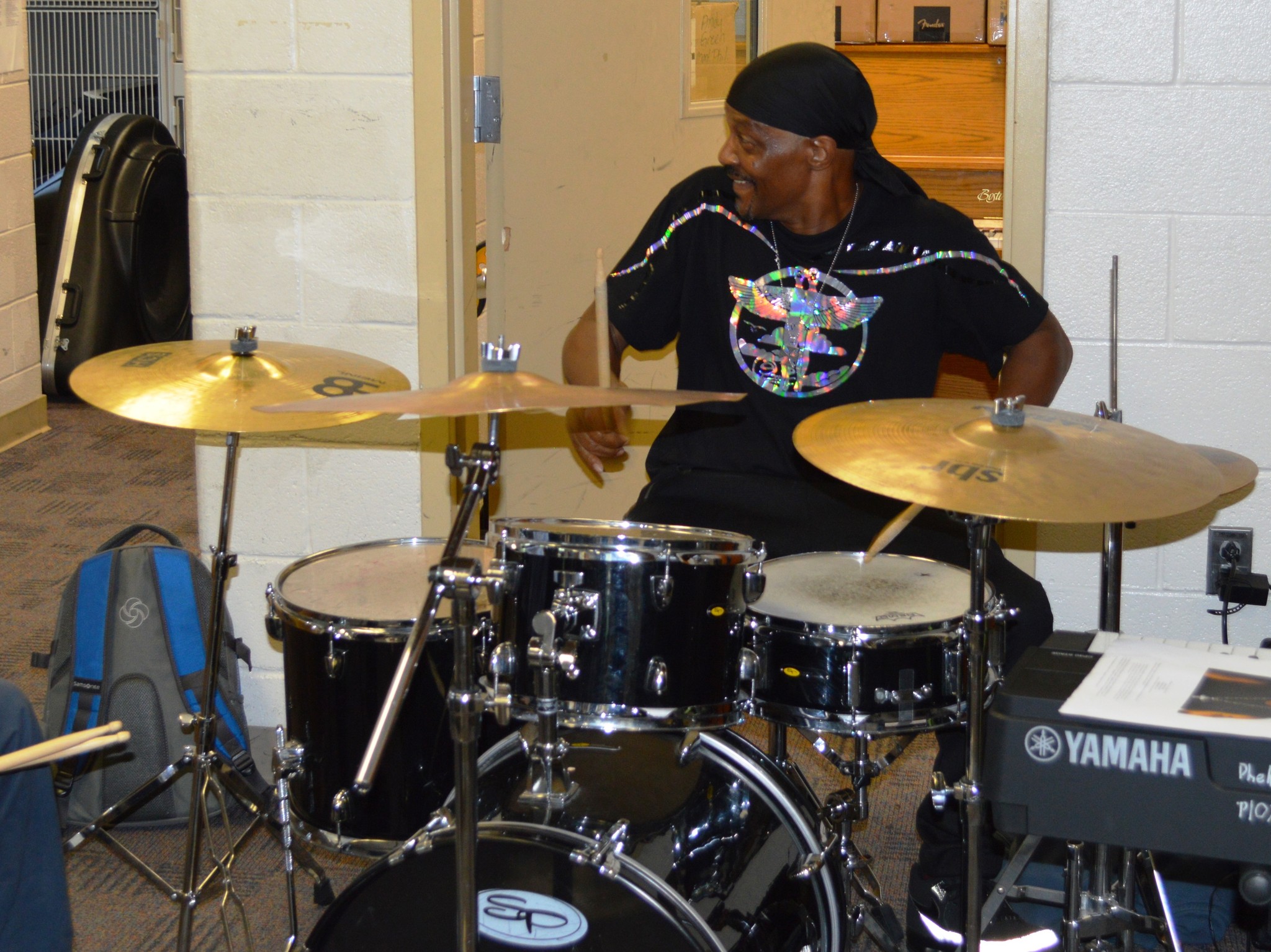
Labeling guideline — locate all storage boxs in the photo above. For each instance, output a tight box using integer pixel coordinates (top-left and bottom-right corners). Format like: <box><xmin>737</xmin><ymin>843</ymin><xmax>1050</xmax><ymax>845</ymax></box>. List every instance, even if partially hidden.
<box><xmin>835</xmin><ymin>0</ymin><xmax>1008</xmax><ymax>46</ymax></box>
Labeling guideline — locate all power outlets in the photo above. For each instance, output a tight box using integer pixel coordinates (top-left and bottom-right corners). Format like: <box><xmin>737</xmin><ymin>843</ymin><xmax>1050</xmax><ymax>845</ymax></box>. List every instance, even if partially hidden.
<box><xmin>1205</xmin><ymin>526</ymin><xmax>1255</xmax><ymax>596</ymax></box>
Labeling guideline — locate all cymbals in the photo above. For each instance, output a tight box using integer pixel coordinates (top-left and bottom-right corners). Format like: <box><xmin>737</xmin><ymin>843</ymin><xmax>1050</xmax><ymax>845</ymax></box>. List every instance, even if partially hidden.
<box><xmin>788</xmin><ymin>395</ymin><xmax>1228</xmax><ymax>529</ymax></box>
<box><xmin>1188</xmin><ymin>443</ymin><xmax>1261</xmax><ymax>494</ymax></box>
<box><xmin>64</xmin><ymin>337</ymin><xmax>413</xmax><ymax>435</ymax></box>
<box><xmin>249</xmin><ymin>370</ymin><xmax>747</xmax><ymax>422</ymax></box>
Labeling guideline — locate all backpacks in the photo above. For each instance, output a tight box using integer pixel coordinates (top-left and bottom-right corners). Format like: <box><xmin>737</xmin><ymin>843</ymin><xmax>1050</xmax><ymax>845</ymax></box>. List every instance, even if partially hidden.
<box><xmin>29</xmin><ymin>523</ymin><xmax>258</xmax><ymax>831</ymax></box>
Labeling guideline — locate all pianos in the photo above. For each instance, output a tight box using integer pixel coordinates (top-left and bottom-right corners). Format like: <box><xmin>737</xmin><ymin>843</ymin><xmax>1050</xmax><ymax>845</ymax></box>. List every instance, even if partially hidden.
<box><xmin>978</xmin><ymin>625</ymin><xmax>1269</xmax><ymax>952</ymax></box>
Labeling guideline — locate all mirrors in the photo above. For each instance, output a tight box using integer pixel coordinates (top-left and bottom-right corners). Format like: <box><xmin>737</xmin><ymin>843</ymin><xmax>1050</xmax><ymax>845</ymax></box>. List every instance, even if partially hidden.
<box><xmin>680</xmin><ymin>0</ymin><xmax>771</xmax><ymax>119</ymax></box>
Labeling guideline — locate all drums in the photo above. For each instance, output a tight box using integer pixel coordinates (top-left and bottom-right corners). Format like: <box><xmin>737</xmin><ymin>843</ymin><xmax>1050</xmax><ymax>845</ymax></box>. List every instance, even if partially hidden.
<box><xmin>474</xmin><ymin>511</ymin><xmax>758</xmax><ymax>738</ymax></box>
<box><xmin>302</xmin><ymin>726</ymin><xmax>852</xmax><ymax>952</ymax></box>
<box><xmin>742</xmin><ymin>548</ymin><xmax>1009</xmax><ymax>741</ymax></box>
<box><xmin>268</xmin><ymin>535</ymin><xmax>533</xmax><ymax>855</ymax></box>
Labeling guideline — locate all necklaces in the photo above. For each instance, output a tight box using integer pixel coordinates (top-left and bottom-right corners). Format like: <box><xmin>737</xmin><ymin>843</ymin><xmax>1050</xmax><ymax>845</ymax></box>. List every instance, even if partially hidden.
<box><xmin>765</xmin><ymin>180</ymin><xmax>856</xmax><ymax>382</ymax></box>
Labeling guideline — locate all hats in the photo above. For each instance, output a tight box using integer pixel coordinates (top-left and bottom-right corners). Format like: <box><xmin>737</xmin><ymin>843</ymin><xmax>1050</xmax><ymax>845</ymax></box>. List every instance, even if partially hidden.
<box><xmin>726</xmin><ymin>42</ymin><xmax>878</xmax><ymax>150</ymax></box>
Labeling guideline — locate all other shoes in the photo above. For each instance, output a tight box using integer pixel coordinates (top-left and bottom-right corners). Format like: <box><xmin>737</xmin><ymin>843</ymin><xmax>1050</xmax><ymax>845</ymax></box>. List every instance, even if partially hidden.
<box><xmin>905</xmin><ymin>886</ymin><xmax>1058</xmax><ymax>952</ymax></box>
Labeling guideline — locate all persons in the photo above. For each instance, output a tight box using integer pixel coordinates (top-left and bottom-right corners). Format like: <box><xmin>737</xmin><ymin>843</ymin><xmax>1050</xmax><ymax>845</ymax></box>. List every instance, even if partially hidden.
<box><xmin>563</xmin><ymin>42</ymin><xmax>1076</xmax><ymax>952</ymax></box>
